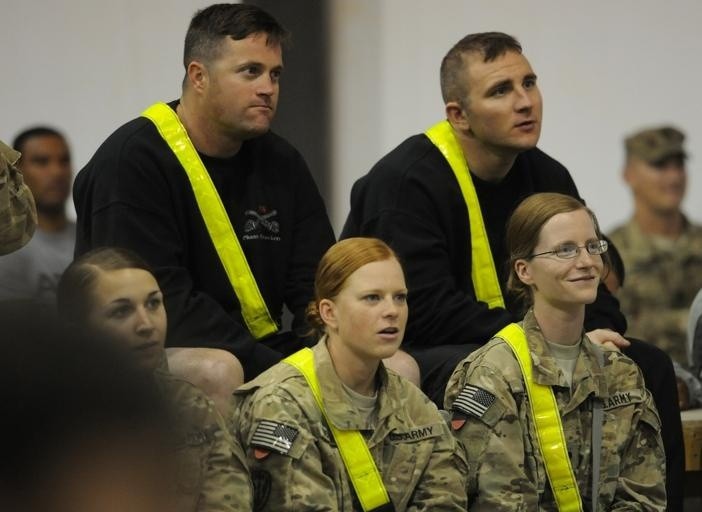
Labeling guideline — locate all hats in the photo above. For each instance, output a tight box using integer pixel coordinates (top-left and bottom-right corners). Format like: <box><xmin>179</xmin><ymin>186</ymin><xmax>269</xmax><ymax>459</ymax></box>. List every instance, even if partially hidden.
<box><xmin>626</xmin><ymin>129</ymin><xmax>686</xmax><ymax>163</ymax></box>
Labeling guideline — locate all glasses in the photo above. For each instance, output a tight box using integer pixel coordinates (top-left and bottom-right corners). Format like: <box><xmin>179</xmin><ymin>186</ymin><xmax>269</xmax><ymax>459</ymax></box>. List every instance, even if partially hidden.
<box><xmin>525</xmin><ymin>240</ymin><xmax>609</xmax><ymax>259</ymax></box>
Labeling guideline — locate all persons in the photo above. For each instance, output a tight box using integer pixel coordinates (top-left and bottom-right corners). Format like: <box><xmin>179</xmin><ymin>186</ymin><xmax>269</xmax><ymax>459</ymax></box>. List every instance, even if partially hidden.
<box><xmin>228</xmin><ymin>236</ymin><xmax>471</xmax><ymax>512</ymax></box>
<box><xmin>603</xmin><ymin>236</ymin><xmax>625</xmax><ymax>297</ymax></box>
<box><xmin>0</xmin><ymin>124</ymin><xmax>198</xmax><ymax>508</ymax></box>
<box><xmin>605</xmin><ymin>128</ymin><xmax>702</xmax><ymax>371</ymax></box>
<box><xmin>443</xmin><ymin>190</ymin><xmax>667</xmax><ymax>512</ymax></box>
<box><xmin>73</xmin><ymin>4</ymin><xmax>421</xmax><ymax>426</ymax></box>
<box><xmin>56</xmin><ymin>244</ymin><xmax>255</xmax><ymax>512</ymax></box>
<box><xmin>338</xmin><ymin>31</ymin><xmax>685</xmax><ymax>512</ymax></box>
